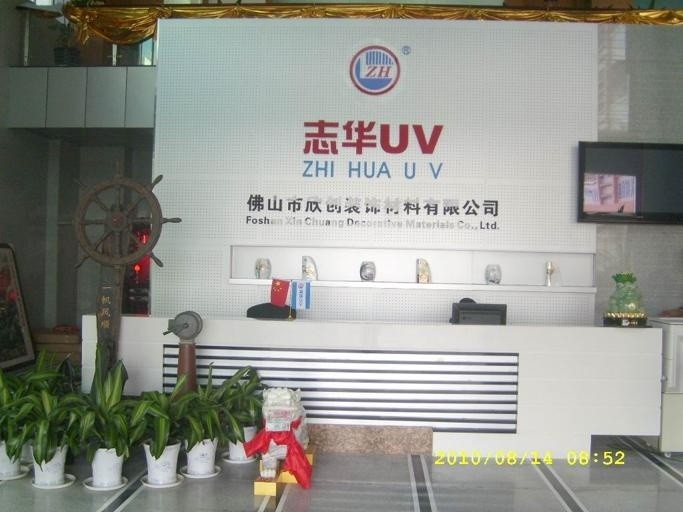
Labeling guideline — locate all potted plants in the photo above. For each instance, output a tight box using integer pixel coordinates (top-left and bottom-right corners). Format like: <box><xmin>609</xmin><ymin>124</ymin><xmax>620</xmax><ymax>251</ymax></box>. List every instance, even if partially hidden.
<box><xmin>180</xmin><ymin>360</ymin><xmax>230</xmax><ymax>479</ymax></box>
<box><xmin>28</xmin><ymin>383</ymin><xmax>77</xmax><ymax>487</ymax></box>
<box><xmin>0</xmin><ymin>365</ymin><xmax>34</xmax><ymax>480</ymax></box>
<box><xmin>216</xmin><ymin>365</ymin><xmax>263</xmax><ymax>462</ymax></box>
<box><xmin>131</xmin><ymin>372</ymin><xmax>186</xmax><ymax>489</ymax></box>
<box><xmin>71</xmin><ymin>342</ymin><xmax>134</xmax><ymax>490</ymax></box>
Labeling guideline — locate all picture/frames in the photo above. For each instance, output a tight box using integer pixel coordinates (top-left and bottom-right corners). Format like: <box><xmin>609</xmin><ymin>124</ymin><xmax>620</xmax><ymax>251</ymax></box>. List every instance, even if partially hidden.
<box><xmin>0</xmin><ymin>241</ymin><xmax>35</xmax><ymax>371</ymax></box>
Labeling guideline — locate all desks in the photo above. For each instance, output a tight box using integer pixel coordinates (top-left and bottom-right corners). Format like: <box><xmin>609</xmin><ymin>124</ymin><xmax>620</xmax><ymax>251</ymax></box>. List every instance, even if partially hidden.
<box><xmin>79</xmin><ymin>317</ymin><xmax>683</xmax><ymax>461</ymax></box>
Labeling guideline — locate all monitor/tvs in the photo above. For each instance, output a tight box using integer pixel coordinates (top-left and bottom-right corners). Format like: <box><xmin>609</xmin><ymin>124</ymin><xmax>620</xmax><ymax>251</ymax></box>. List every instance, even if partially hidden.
<box><xmin>576</xmin><ymin>141</ymin><xmax>683</xmax><ymax>224</ymax></box>
<box><xmin>452</xmin><ymin>303</ymin><xmax>507</xmax><ymax>325</ymax></box>
